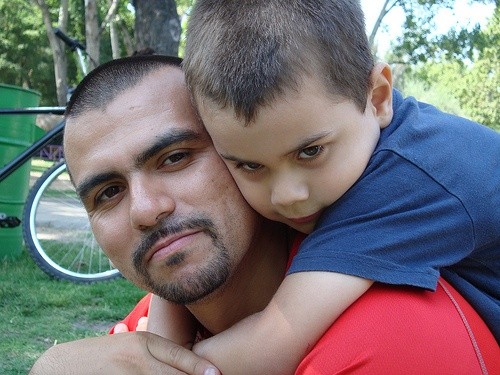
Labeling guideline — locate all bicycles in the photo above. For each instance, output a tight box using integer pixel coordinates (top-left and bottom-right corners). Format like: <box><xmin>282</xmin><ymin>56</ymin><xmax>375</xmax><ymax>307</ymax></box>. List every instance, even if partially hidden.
<box><xmin>0</xmin><ymin>29</ymin><xmax>122</xmax><ymax>283</ymax></box>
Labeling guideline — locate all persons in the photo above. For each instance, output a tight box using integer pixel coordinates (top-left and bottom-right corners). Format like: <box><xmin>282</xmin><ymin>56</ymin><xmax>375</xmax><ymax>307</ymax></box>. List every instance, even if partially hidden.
<box><xmin>27</xmin><ymin>55</ymin><xmax>500</xmax><ymax>375</ymax></box>
<box><xmin>145</xmin><ymin>0</ymin><xmax>500</xmax><ymax>375</ymax></box>
